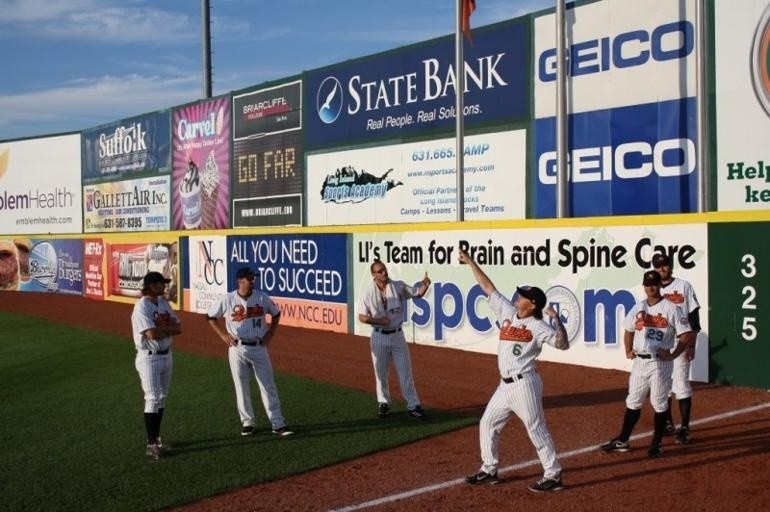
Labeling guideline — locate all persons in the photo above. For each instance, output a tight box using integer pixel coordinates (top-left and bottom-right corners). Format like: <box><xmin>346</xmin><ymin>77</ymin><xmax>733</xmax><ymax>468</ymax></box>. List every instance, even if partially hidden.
<box><xmin>358</xmin><ymin>260</ymin><xmax>431</xmax><ymax>419</ymax></box>
<box><xmin>653</xmin><ymin>253</ymin><xmax>701</xmax><ymax>446</ymax></box>
<box><xmin>599</xmin><ymin>270</ymin><xmax>692</xmax><ymax>458</ymax></box>
<box><xmin>457</xmin><ymin>247</ymin><xmax>569</xmax><ymax>495</ymax></box>
<box><xmin>131</xmin><ymin>272</ymin><xmax>182</xmax><ymax>459</ymax></box>
<box><xmin>204</xmin><ymin>267</ymin><xmax>294</xmax><ymax>437</ymax></box>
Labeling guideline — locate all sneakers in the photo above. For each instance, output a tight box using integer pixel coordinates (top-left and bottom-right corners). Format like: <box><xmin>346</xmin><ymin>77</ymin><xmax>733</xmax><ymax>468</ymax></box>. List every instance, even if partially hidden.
<box><xmin>528</xmin><ymin>477</ymin><xmax>564</xmax><ymax>492</ymax></box>
<box><xmin>409</xmin><ymin>406</ymin><xmax>425</xmax><ymax>417</ymax></box>
<box><xmin>664</xmin><ymin>424</ymin><xmax>692</xmax><ymax>444</ymax></box>
<box><xmin>241</xmin><ymin>427</ymin><xmax>255</xmax><ymax>436</ymax></box>
<box><xmin>466</xmin><ymin>471</ymin><xmax>497</xmax><ymax>483</ymax></box>
<box><xmin>601</xmin><ymin>440</ymin><xmax>629</xmax><ymax>452</ymax></box>
<box><xmin>648</xmin><ymin>445</ymin><xmax>663</xmax><ymax>457</ymax></box>
<box><xmin>147</xmin><ymin>437</ymin><xmax>171</xmax><ymax>459</ymax></box>
<box><xmin>378</xmin><ymin>403</ymin><xmax>391</xmax><ymax>416</ymax></box>
<box><xmin>272</xmin><ymin>427</ymin><xmax>294</xmax><ymax>436</ymax></box>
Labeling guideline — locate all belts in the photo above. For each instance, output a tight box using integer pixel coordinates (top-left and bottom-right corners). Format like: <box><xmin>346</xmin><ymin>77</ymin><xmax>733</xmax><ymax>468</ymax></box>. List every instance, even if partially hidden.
<box><xmin>503</xmin><ymin>374</ymin><xmax>523</xmax><ymax>383</ymax></box>
<box><xmin>636</xmin><ymin>354</ymin><xmax>659</xmax><ymax>358</ymax></box>
<box><xmin>376</xmin><ymin>328</ymin><xmax>401</xmax><ymax>334</ymax></box>
<box><xmin>234</xmin><ymin>340</ymin><xmax>262</xmax><ymax>346</ymax></box>
<box><xmin>150</xmin><ymin>348</ymin><xmax>169</xmax><ymax>354</ymax></box>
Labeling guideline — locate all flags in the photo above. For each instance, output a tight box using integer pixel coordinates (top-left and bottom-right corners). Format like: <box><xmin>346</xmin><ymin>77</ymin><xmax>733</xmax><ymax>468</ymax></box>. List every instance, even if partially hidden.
<box><xmin>460</xmin><ymin>1</ymin><xmax>476</xmax><ymax>47</ymax></box>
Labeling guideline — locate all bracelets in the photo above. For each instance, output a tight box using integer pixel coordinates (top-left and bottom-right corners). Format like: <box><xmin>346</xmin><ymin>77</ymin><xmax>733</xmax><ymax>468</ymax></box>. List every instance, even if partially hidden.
<box><xmin>266</xmin><ymin>331</ymin><xmax>273</xmax><ymax>336</ymax></box>
<box><xmin>688</xmin><ymin>344</ymin><xmax>695</xmax><ymax>347</ymax></box>
<box><xmin>552</xmin><ymin>312</ymin><xmax>558</xmax><ymax>317</ymax></box>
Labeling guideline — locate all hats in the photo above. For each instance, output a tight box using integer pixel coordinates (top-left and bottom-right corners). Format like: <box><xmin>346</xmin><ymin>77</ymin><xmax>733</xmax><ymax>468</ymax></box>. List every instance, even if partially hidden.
<box><xmin>144</xmin><ymin>272</ymin><xmax>171</xmax><ymax>283</ymax></box>
<box><xmin>654</xmin><ymin>255</ymin><xmax>670</xmax><ymax>267</ymax></box>
<box><xmin>517</xmin><ymin>287</ymin><xmax>546</xmax><ymax>308</ymax></box>
<box><xmin>644</xmin><ymin>271</ymin><xmax>661</xmax><ymax>286</ymax></box>
<box><xmin>237</xmin><ymin>269</ymin><xmax>260</xmax><ymax>278</ymax></box>
